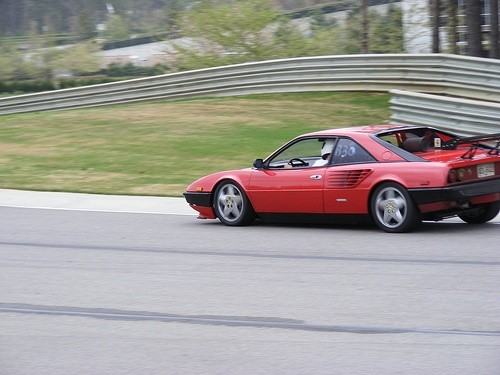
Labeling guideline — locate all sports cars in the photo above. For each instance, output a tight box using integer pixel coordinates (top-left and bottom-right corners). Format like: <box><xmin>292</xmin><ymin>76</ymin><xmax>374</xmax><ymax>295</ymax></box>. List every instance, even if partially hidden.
<box><xmin>182</xmin><ymin>121</ymin><xmax>500</xmax><ymax>235</ymax></box>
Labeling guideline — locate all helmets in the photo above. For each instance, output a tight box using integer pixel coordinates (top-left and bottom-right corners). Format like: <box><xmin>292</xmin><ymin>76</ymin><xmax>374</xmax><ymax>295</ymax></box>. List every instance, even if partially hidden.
<box><xmin>321</xmin><ymin>139</ymin><xmax>336</xmax><ymax>159</ymax></box>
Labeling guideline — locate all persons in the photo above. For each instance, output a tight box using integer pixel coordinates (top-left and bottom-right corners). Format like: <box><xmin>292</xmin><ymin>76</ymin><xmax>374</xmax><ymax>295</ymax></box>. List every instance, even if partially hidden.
<box><xmin>282</xmin><ymin>139</ymin><xmax>337</xmax><ymax>172</ymax></box>
